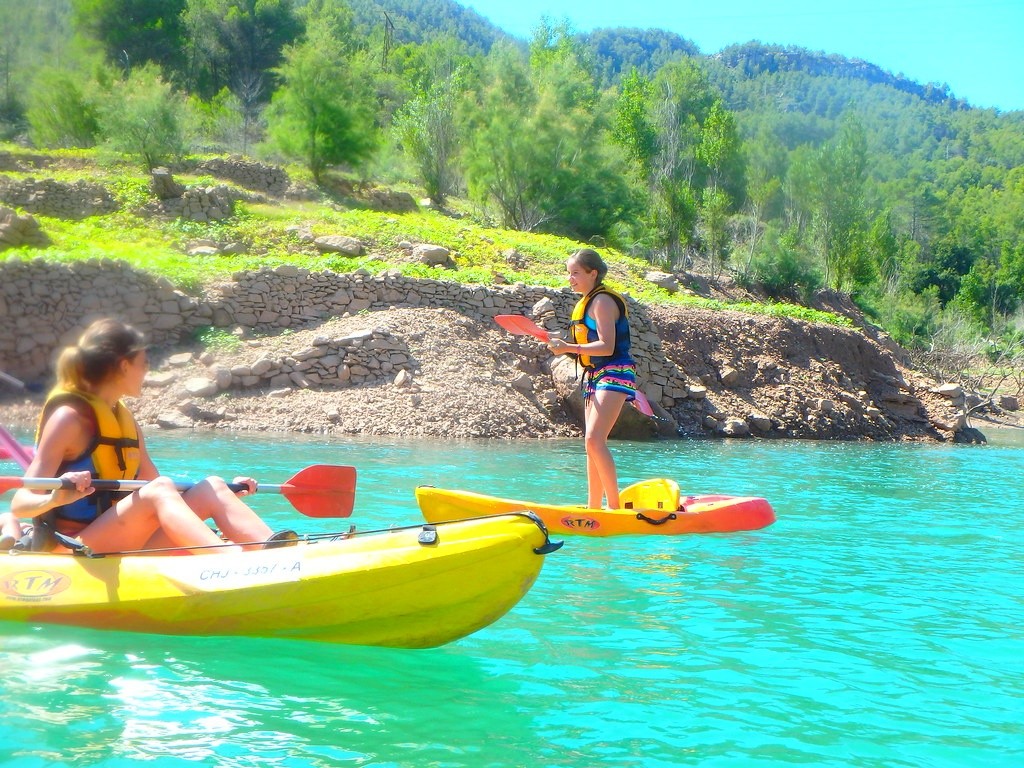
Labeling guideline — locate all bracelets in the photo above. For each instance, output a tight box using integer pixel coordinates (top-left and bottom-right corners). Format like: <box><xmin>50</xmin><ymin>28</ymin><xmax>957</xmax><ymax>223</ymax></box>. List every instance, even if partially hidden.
<box><xmin>576</xmin><ymin>344</ymin><xmax>581</xmax><ymax>355</ymax></box>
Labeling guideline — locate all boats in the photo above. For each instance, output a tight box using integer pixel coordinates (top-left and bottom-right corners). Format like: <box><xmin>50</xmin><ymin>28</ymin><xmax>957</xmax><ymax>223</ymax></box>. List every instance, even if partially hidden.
<box><xmin>415</xmin><ymin>477</ymin><xmax>777</xmax><ymax>539</ymax></box>
<box><xmin>0</xmin><ymin>511</ymin><xmax>565</xmax><ymax>652</ymax></box>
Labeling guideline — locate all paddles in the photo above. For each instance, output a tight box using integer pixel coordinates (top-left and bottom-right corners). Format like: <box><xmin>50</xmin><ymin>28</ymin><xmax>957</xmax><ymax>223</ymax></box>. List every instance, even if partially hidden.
<box><xmin>1</xmin><ymin>370</ymin><xmax>43</xmax><ymax>394</ymax></box>
<box><xmin>1</xmin><ymin>460</ymin><xmax>360</xmax><ymax>521</ymax></box>
<box><xmin>493</xmin><ymin>313</ymin><xmax>656</xmax><ymax>418</ymax></box>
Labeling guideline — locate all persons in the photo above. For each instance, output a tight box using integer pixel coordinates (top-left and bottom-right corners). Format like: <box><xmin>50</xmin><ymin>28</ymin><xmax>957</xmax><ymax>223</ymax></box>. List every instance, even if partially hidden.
<box><xmin>547</xmin><ymin>249</ymin><xmax>636</xmax><ymax>511</ymax></box>
<box><xmin>0</xmin><ymin>512</ymin><xmax>23</xmax><ymax>559</ymax></box>
<box><xmin>11</xmin><ymin>317</ymin><xmax>300</xmax><ymax>558</ymax></box>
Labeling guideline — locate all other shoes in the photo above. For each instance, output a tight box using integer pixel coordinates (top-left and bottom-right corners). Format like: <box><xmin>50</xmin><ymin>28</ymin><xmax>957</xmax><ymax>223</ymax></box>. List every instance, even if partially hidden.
<box><xmin>263</xmin><ymin>529</ymin><xmax>299</xmax><ymax>549</ymax></box>
